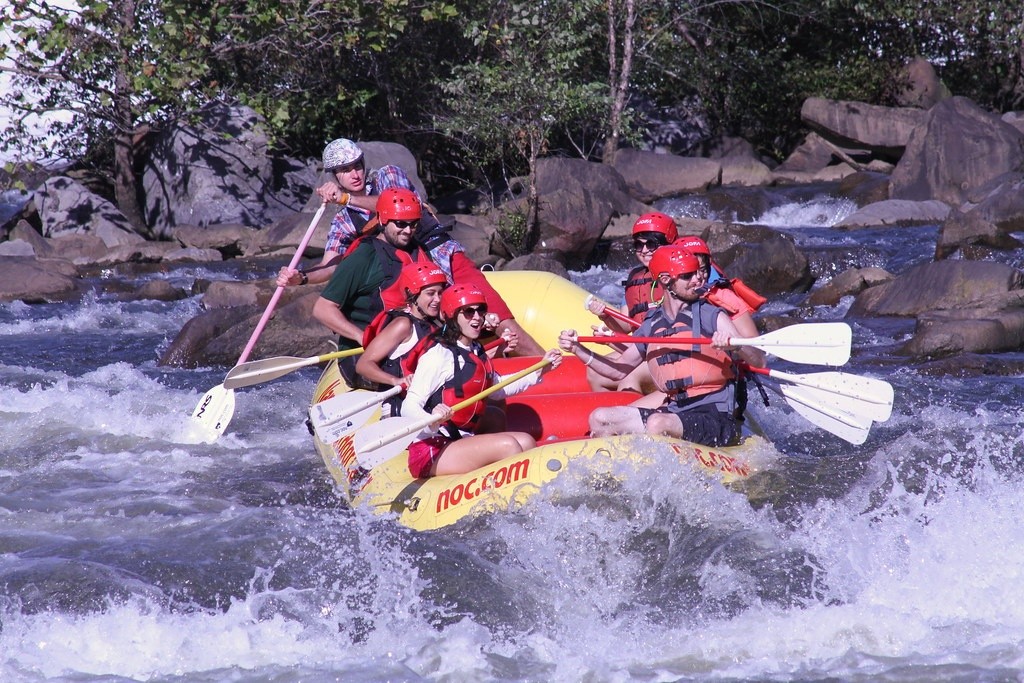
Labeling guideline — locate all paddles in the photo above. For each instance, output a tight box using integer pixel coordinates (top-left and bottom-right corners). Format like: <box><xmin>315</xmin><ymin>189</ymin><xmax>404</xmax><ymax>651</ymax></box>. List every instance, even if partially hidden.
<box><xmin>574</xmin><ymin>322</ymin><xmax>854</xmax><ymax>368</ymax></box>
<box><xmin>223</xmin><ymin>315</ymin><xmax>495</xmax><ymax>391</ymax></box>
<box><xmin>189</xmin><ymin>195</ymin><xmax>336</xmax><ymax>441</ymax></box>
<box><xmin>596</xmin><ymin>323</ymin><xmax>872</xmax><ymax>447</ymax></box>
<box><xmin>585</xmin><ymin>294</ymin><xmax>894</xmax><ymax>424</ymax></box>
<box><xmin>310</xmin><ymin>328</ymin><xmax>510</xmax><ymax>446</ymax></box>
<box><xmin>353</xmin><ymin>353</ymin><xmax>555</xmax><ymax>471</ymax></box>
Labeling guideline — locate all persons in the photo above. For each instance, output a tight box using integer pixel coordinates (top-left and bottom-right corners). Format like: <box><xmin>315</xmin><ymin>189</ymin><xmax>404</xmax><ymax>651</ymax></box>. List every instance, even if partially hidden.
<box><xmin>273</xmin><ymin>138</ymin><xmax>769</xmax><ymax>477</ymax></box>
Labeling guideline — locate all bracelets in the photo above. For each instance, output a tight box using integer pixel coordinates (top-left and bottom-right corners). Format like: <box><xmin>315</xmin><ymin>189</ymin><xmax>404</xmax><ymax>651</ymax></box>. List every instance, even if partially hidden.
<box><xmin>584</xmin><ymin>349</ymin><xmax>595</xmax><ymax>367</ymax></box>
<box><xmin>299</xmin><ymin>270</ymin><xmax>308</xmax><ymax>285</ymax></box>
<box><xmin>340</xmin><ymin>191</ymin><xmax>349</xmax><ymax>206</ymax></box>
<box><xmin>346</xmin><ymin>193</ymin><xmax>351</xmax><ymax>206</ymax></box>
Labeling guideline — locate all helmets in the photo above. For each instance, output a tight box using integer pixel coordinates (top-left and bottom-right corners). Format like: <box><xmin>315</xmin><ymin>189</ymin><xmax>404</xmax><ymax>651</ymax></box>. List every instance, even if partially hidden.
<box><xmin>400</xmin><ymin>262</ymin><xmax>447</xmax><ymax>303</ymax></box>
<box><xmin>376</xmin><ymin>188</ymin><xmax>423</xmax><ymax>225</ymax></box>
<box><xmin>322</xmin><ymin>138</ymin><xmax>364</xmax><ymax>171</ymax></box>
<box><xmin>672</xmin><ymin>236</ymin><xmax>710</xmax><ymax>255</ymax></box>
<box><xmin>649</xmin><ymin>245</ymin><xmax>699</xmax><ymax>280</ymax></box>
<box><xmin>632</xmin><ymin>213</ymin><xmax>678</xmax><ymax>244</ymax></box>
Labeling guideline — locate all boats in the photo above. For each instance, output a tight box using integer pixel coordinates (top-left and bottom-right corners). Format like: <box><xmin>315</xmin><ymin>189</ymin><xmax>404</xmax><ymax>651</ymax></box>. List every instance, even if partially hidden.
<box><xmin>307</xmin><ymin>271</ymin><xmax>767</xmax><ymax>532</ymax></box>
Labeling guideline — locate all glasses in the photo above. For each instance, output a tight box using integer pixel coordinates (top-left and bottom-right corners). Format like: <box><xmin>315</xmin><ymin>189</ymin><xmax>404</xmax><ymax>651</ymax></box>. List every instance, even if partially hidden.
<box><xmin>390</xmin><ymin>220</ymin><xmax>420</xmax><ymax>228</ymax></box>
<box><xmin>440</xmin><ymin>283</ymin><xmax>488</xmax><ymax>318</ymax></box>
<box><xmin>679</xmin><ymin>272</ymin><xmax>697</xmax><ymax>279</ymax></box>
<box><xmin>633</xmin><ymin>239</ymin><xmax>660</xmax><ymax>252</ymax></box>
<box><xmin>458</xmin><ymin>305</ymin><xmax>486</xmax><ymax>319</ymax></box>
